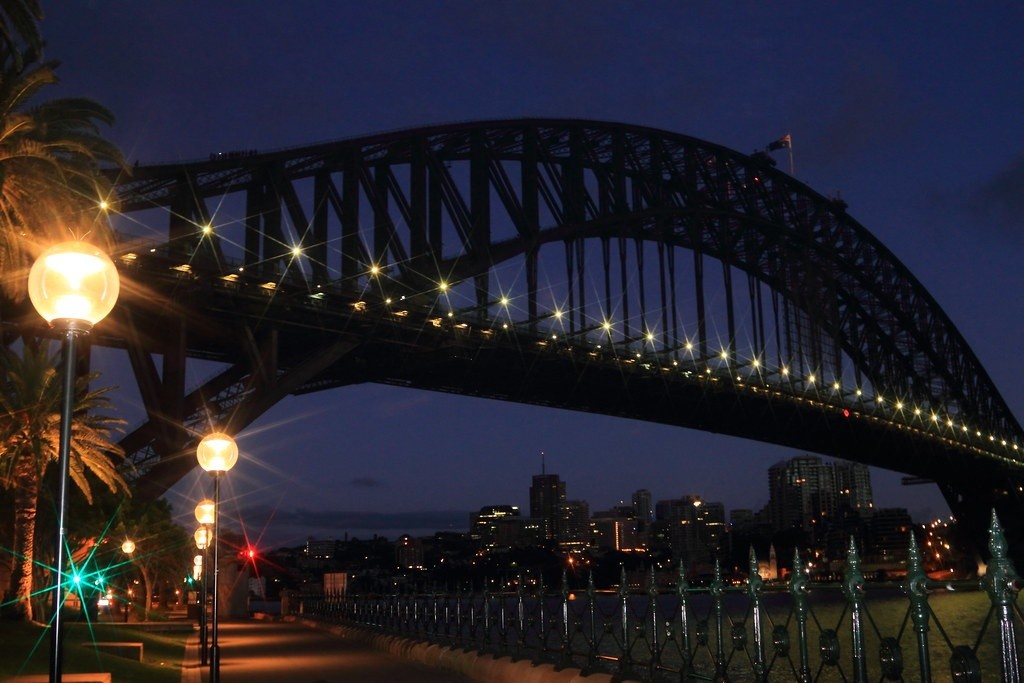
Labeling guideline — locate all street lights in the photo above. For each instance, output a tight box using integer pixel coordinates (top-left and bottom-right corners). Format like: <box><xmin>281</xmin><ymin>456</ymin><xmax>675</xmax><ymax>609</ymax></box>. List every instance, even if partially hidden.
<box><xmin>195</xmin><ymin>432</ymin><xmax>238</xmax><ymax>683</ymax></box>
<box><xmin>122</xmin><ymin>539</ymin><xmax>137</xmax><ymax>619</ymax></box>
<box><xmin>189</xmin><ymin>500</ymin><xmax>215</xmax><ymax>668</ymax></box>
<box><xmin>25</xmin><ymin>239</ymin><xmax>121</xmax><ymax>682</ymax></box>
